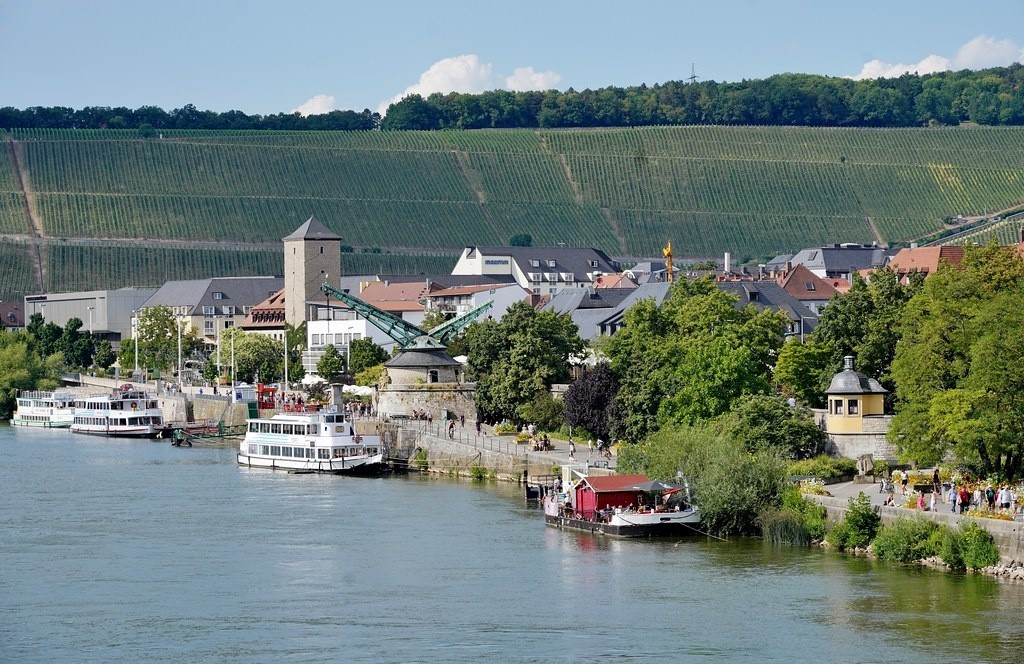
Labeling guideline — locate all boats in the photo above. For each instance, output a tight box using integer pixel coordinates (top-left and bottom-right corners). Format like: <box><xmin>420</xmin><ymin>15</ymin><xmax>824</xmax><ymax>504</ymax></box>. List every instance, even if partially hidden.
<box><xmin>543</xmin><ymin>473</ymin><xmax>702</xmax><ymax>540</ymax></box>
<box><xmin>10</xmin><ymin>388</ymin><xmax>75</xmax><ymax>429</ymax></box>
<box><xmin>235</xmin><ymin>409</ymin><xmax>389</xmax><ymax>482</ymax></box>
<box><xmin>67</xmin><ymin>385</ymin><xmax>164</xmax><ymax>439</ymax></box>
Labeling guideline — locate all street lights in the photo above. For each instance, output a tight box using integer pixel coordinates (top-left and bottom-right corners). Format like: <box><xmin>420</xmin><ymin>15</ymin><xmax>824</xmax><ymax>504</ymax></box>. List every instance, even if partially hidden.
<box><xmin>132</xmin><ymin>309</ymin><xmax>143</xmax><ymax>372</ymax></box>
<box><xmin>87</xmin><ymin>307</ymin><xmax>94</xmax><ymax>337</ymax></box>
<box><xmin>284</xmin><ymin>329</ymin><xmax>291</xmax><ymax>391</ymax></box>
<box><xmin>39</xmin><ymin>303</ymin><xmax>46</xmax><ymax>319</ymax></box>
<box><xmin>231</xmin><ymin>331</ymin><xmax>238</xmax><ymax>389</ymax></box>
<box><xmin>174</xmin><ymin>311</ymin><xmax>184</xmax><ymax>381</ymax></box>
<box><xmin>213</xmin><ymin>315</ymin><xmax>223</xmax><ymax>388</ymax></box>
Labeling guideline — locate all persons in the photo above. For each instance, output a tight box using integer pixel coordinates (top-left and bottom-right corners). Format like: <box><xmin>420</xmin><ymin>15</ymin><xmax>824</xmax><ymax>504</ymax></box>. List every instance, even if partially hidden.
<box><xmin>900</xmin><ymin>468</ymin><xmax>909</xmax><ymax>496</ymax></box>
<box><xmin>930</xmin><ymin>488</ymin><xmax>938</xmax><ymax>512</ymax></box>
<box><xmin>412</xmin><ymin>407</ymin><xmax>616</xmax><ymax>462</ymax></box>
<box><xmin>88</xmin><ymin>366</ymin><xmax>243</xmax><ymax>412</ymax></box>
<box><xmin>940</xmin><ymin>468</ymin><xmax>1024</xmax><ymax>522</ymax></box>
<box><xmin>887</xmin><ymin>492</ymin><xmax>901</xmax><ymax>508</ymax></box>
<box><xmin>932</xmin><ymin>469</ymin><xmax>940</xmax><ymax>492</ymax></box>
<box><xmin>542</xmin><ymin>474</ymin><xmax>686</xmax><ymax>524</ymax></box>
<box><xmin>878</xmin><ymin>471</ymin><xmax>924</xmax><ymax>493</ymax></box>
<box><xmin>917</xmin><ymin>492</ymin><xmax>930</xmax><ymax>511</ymax></box>
<box><xmin>260</xmin><ymin>383</ymin><xmax>377</xmax><ymax>419</ymax></box>
